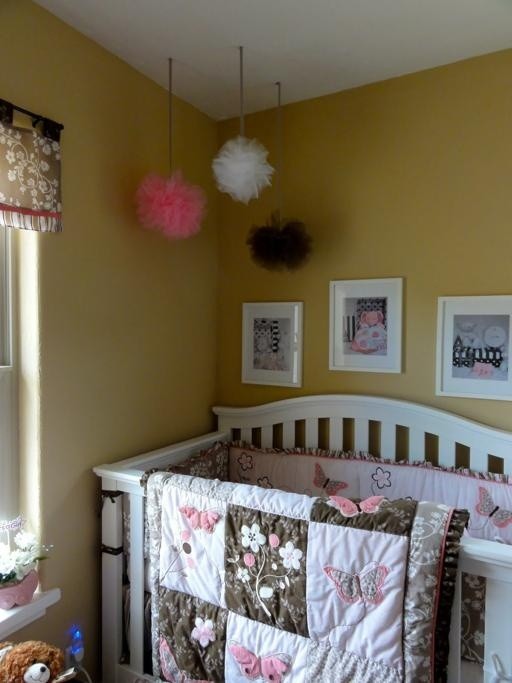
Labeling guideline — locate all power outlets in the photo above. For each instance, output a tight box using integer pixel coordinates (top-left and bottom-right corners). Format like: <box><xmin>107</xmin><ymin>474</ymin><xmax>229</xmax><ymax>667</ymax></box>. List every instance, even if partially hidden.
<box><xmin>65</xmin><ymin>645</ymin><xmax>82</xmax><ymax>670</ymax></box>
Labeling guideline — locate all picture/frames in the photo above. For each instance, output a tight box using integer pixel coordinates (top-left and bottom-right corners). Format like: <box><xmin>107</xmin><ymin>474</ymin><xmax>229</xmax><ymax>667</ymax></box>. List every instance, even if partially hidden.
<box><xmin>435</xmin><ymin>296</ymin><xmax>512</xmax><ymax>401</ymax></box>
<box><xmin>328</xmin><ymin>277</ymin><xmax>403</xmax><ymax>373</ymax></box>
<box><xmin>241</xmin><ymin>301</ymin><xmax>303</xmax><ymax>388</ymax></box>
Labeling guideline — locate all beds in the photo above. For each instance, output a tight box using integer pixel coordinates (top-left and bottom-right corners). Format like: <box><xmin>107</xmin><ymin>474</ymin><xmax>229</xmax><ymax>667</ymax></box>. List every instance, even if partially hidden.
<box><xmin>91</xmin><ymin>393</ymin><xmax>512</xmax><ymax>683</ymax></box>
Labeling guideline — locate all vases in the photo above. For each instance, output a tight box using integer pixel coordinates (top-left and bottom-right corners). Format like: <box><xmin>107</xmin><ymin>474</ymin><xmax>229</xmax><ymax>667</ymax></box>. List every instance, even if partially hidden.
<box><xmin>0</xmin><ymin>569</ymin><xmax>39</xmax><ymax>610</ymax></box>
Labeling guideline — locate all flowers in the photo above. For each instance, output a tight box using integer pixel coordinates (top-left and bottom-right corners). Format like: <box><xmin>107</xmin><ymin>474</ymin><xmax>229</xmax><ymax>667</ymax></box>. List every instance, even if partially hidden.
<box><xmin>0</xmin><ymin>516</ymin><xmax>54</xmax><ymax>580</ymax></box>
